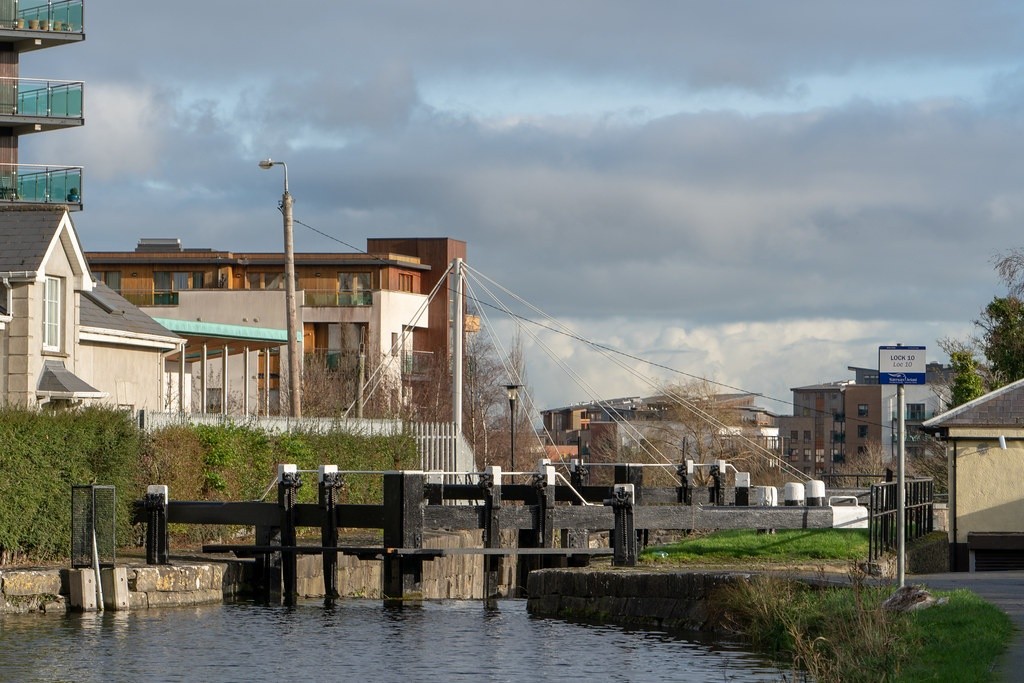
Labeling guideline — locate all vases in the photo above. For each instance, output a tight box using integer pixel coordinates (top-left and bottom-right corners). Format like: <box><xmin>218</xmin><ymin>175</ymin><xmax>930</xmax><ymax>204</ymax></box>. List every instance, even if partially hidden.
<box><xmin>14</xmin><ymin>19</ymin><xmax>25</xmax><ymax>29</ymax></box>
<box><xmin>28</xmin><ymin>19</ymin><xmax>64</xmax><ymax>32</ymax></box>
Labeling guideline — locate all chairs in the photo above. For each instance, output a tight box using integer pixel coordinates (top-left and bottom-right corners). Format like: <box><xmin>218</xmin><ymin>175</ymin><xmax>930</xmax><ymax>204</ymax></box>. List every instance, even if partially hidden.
<box><xmin>1</xmin><ymin>176</ymin><xmax>17</xmax><ymax>200</ymax></box>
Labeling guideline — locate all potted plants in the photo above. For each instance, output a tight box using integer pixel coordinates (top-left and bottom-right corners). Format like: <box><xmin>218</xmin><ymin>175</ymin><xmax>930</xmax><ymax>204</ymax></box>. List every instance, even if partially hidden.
<box><xmin>67</xmin><ymin>187</ymin><xmax>79</xmax><ymax>202</ymax></box>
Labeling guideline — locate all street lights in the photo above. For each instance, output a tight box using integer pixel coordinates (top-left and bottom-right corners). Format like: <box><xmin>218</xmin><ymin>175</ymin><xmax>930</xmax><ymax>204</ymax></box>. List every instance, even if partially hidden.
<box><xmin>258</xmin><ymin>157</ymin><xmax>302</xmax><ymax>416</ymax></box>
<box><xmin>500</xmin><ymin>382</ymin><xmax>525</xmax><ymax>485</ymax></box>
